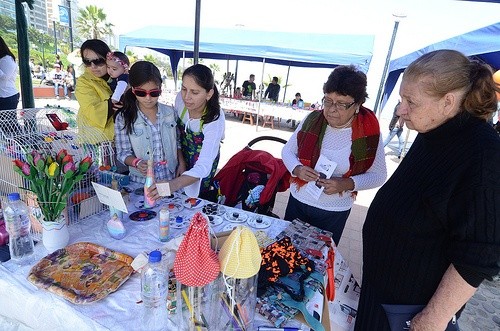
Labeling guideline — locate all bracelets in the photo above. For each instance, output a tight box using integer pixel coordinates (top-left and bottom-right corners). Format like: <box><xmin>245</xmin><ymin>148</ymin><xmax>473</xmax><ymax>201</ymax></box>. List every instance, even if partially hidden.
<box><xmin>131</xmin><ymin>158</ymin><xmax>144</xmax><ymax>168</ymax></box>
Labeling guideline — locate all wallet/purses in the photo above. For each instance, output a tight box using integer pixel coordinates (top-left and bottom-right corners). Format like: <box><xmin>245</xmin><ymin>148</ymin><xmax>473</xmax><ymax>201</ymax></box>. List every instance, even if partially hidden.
<box><xmin>381</xmin><ymin>304</ymin><xmax>460</xmax><ymax>331</ymax></box>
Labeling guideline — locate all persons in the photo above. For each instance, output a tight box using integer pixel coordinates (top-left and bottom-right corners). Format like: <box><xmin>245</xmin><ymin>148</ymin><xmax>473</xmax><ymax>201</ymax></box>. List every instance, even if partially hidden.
<box><xmin>29</xmin><ymin>54</ymin><xmax>75</xmax><ymax>101</ymax></box>
<box><xmin>0</xmin><ymin>36</ymin><xmax>24</xmax><ymax>149</ymax></box>
<box><xmin>383</xmin><ymin>98</ymin><xmax>405</xmax><ymax>159</ymax></box>
<box><xmin>286</xmin><ymin>92</ymin><xmax>303</xmax><ymax>129</ymax></box>
<box><xmin>241</xmin><ymin>74</ymin><xmax>256</xmax><ymax>99</ymax></box>
<box><xmin>282</xmin><ymin>62</ymin><xmax>387</xmax><ymax>247</ymax></box>
<box><xmin>75</xmin><ymin>39</ymin><xmax>225</xmax><ymax>201</ymax></box>
<box><xmin>263</xmin><ymin>77</ymin><xmax>280</xmax><ymax>121</ymax></box>
<box><xmin>354</xmin><ymin>49</ymin><xmax>500</xmax><ymax>331</ymax></box>
<box><xmin>234</xmin><ymin>87</ymin><xmax>242</xmax><ymax>117</ymax></box>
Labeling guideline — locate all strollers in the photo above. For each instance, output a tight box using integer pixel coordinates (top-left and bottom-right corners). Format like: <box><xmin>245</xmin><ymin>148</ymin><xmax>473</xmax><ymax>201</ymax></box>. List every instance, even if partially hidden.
<box><xmin>207</xmin><ymin>136</ymin><xmax>294</xmax><ymax>221</ymax></box>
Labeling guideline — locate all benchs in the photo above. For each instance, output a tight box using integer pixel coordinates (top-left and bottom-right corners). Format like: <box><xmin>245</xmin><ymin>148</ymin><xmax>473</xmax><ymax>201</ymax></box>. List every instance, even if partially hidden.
<box><xmin>34</xmin><ymin>84</ymin><xmax>71</xmax><ymax>98</ymax></box>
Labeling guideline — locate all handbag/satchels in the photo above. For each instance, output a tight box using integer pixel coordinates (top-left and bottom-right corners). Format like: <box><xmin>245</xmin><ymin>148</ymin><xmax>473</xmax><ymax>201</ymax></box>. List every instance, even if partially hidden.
<box><xmin>257</xmin><ymin>236</ymin><xmax>331</xmax><ymax>331</ymax></box>
<box><xmin>218</xmin><ymin>224</ymin><xmax>262</xmax><ymax>279</ymax></box>
<box><xmin>173</xmin><ymin>211</ymin><xmax>220</xmax><ymax>287</ymax></box>
<box><xmin>276</xmin><ymin>218</ymin><xmax>335</xmax><ymax>301</ymax></box>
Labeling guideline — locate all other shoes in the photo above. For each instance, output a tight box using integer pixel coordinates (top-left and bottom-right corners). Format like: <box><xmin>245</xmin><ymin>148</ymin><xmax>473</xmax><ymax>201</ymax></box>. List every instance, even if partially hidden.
<box><xmin>56</xmin><ymin>96</ymin><xmax>60</xmax><ymax>100</ymax></box>
<box><xmin>65</xmin><ymin>96</ymin><xmax>70</xmax><ymax>100</ymax></box>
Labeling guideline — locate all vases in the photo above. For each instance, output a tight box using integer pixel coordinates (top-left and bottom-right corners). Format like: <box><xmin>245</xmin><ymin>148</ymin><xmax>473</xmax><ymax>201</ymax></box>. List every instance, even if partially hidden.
<box><xmin>42</xmin><ymin>215</ymin><xmax>69</xmax><ymax>251</ymax></box>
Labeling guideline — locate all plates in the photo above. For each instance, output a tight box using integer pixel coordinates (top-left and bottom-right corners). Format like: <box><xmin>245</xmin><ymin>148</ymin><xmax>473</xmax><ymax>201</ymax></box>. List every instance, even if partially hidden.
<box><xmin>182</xmin><ymin>197</ymin><xmax>205</xmax><ymax>209</ymax></box>
<box><xmin>206</xmin><ymin>215</ymin><xmax>224</xmax><ymax>226</ymax></box>
<box><xmin>200</xmin><ymin>203</ymin><xmax>227</xmax><ymax>216</ymax></box>
<box><xmin>159</xmin><ymin>192</ymin><xmax>183</xmax><ymax>203</ymax></box>
<box><xmin>168</xmin><ymin>215</ymin><xmax>190</xmax><ymax>228</ymax></box>
<box><xmin>224</xmin><ymin>211</ymin><xmax>248</xmax><ymax>222</ymax></box>
<box><xmin>158</xmin><ymin>203</ymin><xmax>184</xmax><ymax>214</ymax></box>
<box><xmin>247</xmin><ymin>216</ymin><xmax>271</xmax><ymax>228</ymax></box>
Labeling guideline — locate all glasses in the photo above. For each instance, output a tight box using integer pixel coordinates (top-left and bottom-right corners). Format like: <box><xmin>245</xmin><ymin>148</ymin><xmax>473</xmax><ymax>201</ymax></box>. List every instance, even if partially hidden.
<box><xmin>83</xmin><ymin>58</ymin><xmax>106</xmax><ymax>67</ymax></box>
<box><xmin>132</xmin><ymin>86</ymin><xmax>162</xmax><ymax>97</ymax></box>
<box><xmin>322</xmin><ymin>96</ymin><xmax>355</xmax><ymax>110</ymax></box>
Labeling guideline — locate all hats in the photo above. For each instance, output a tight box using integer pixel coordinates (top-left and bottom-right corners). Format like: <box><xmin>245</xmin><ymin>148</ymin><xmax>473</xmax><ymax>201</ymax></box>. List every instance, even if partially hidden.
<box><xmin>54</xmin><ymin>64</ymin><xmax>60</xmax><ymax>68</ymax></box>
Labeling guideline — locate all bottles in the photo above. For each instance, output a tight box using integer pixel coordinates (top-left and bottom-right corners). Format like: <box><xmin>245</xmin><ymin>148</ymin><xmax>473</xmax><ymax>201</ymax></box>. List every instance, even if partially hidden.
<box><xmin>144</xmin><ymin>160</ymin><xmax>156</xmax><ymax>208</ymax></box>
<box><xmin>139</xmin><ymin>250</ymin><xmax>169</xmax><ymax>331</ymax></box>
<box><xmin>108</xmin><ymin>180</ymin><xmax>126</xmax><ymax>240</ymax></box>
<box><xmin>5</xmin><ymin>194</ymin><xmax>36</xmax><ymax>266</ymax></box>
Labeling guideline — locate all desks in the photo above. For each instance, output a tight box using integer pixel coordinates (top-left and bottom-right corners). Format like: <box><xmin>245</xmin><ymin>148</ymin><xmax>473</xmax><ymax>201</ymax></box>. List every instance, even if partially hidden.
<box><xmin>0</xmin><ymin>191</ymin><xmax>362</xmax><ymax>331</ymax></box>
<box><xmin>221</xmin><ymin>97</ymin><xmax>312</xmax><ymax>128</ymax></box>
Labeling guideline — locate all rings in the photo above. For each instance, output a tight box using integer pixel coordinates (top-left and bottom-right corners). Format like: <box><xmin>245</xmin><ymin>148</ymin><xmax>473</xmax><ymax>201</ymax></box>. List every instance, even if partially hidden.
<box><xmin>303</xmin><ymin>178</ymin><xmax>305</xmax><ymax>180</ymax></box>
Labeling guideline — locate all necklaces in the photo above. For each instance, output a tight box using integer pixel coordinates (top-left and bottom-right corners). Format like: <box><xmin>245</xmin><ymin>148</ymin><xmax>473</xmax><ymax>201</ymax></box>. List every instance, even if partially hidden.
<box><xmin>335</xmin><ymin>115</ymin><xmax>354</xmax><ymax>129</ymax></box>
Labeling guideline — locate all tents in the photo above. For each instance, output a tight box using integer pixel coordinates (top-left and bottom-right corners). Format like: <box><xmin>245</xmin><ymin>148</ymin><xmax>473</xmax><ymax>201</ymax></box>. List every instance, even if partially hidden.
<box><xmin>119</xmin><ymin>24</ymin><xmax>375</xmax><ymax>91</ymax></box>
<box><xmin>379</xmin><ymin>0</ymin><xmax>500</xmax><ymax>118</ymax></box>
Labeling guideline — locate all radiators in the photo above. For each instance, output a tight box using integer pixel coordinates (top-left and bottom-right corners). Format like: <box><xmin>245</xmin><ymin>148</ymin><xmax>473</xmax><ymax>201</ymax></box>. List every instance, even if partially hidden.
<box><xmin>13</xmin><ymin>149</ymin><xmax>95</xmax><ymax>222</ymax></box>
